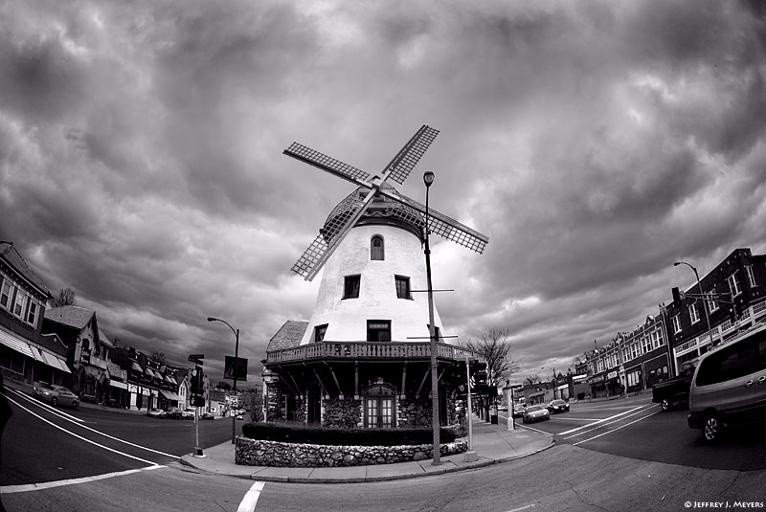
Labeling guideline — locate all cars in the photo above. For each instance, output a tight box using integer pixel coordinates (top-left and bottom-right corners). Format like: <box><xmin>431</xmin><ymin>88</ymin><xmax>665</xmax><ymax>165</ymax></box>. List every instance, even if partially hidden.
<box><xmin>235</xmin><ymin>409</ymin><xmax>246</xmax><ymax>420</ymax></box>
<box><xmin>653</xmin><ymin>323</ymin><xmax>766</xmax><ymax>438</ymax></box>
<box><xmin>497</xmin><ymin>399</ymin><xmax>570</xmax><ymax>424</ymax></box>
<box><xmin>32</xmin><ymin>381</ymin><xmax>80</xmax><ymax>410</ymax></box>
<box><xmin>148</xmin><ymin>407</ymin><xmax>214</xmax><ymax>421</ymax></box>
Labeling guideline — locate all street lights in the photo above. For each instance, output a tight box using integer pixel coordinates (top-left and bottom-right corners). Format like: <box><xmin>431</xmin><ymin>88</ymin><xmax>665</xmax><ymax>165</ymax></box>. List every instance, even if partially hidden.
<box><xmin>673</xmin><ymin>260</ymin><xmax>715</xmax><ymax>346</ymax></box>
<box><xmin>207</xmin><ymin>317</ymin><xmax>240</xmax><ymax>444</ymax></box>
<box><xmin>423</xmin><ymin>170</ymin><xmax>440</xmax><ymax>464</ymax></box>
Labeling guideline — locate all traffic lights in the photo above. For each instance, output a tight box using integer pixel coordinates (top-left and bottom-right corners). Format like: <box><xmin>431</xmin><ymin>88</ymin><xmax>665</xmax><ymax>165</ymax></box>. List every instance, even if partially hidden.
<box><xmin>475</xmin><ymin>359</ymin><xmax>489</xmax><ymax>394</ymax></box>
<box><xmin>191</xmin><ymin>367</ymin><xmax>205</xmax><ymax>398</ymax></box>
<box><xmin>671</xmin><ymin>287</ymin><xmax>681</xmax><ymax>306</ymax></box>
